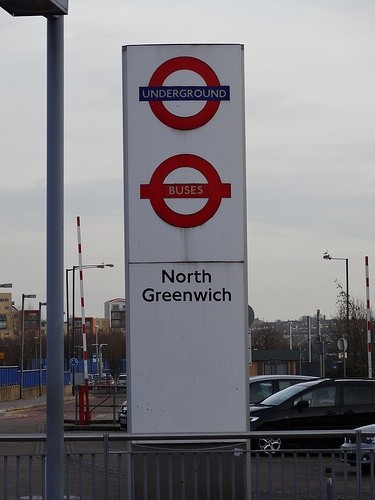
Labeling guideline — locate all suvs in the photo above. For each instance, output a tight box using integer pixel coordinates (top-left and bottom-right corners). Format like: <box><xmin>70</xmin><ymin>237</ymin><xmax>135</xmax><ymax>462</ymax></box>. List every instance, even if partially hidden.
<box><xmin>249</xmin><ymin>377</ymin><xmax>375</xmax><ymax>458</ymax></box>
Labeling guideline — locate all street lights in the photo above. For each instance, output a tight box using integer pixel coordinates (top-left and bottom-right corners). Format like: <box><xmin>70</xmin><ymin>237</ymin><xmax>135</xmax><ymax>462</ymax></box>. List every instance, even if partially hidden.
<box><xmin>19</xmin><ymin>294</ymin><xmax>37</xmax><ymax>399</ymax></box>
<box><xmin>66</xmin><ymin>266</ymin><xmax>105</xmax><ymax>372</ymax></box>
<box><xmin>323</xmin><ymin>255</ymin><xmax>349</xmax><ymax>377</ymax></box>
<box><xmin>0</xmin><ymin>0</ymin><xmax>68</xmax><ymax>500</ymax></box>
<box><xmin>73</xmin><ymin>264</ymin><xmax>114</xmax><ymax>396</ymax></box>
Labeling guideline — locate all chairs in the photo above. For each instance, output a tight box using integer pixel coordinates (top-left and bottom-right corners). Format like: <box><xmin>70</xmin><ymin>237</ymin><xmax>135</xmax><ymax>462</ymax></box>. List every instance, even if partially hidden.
<box><xmin>310</xmin><ymin>389</ymin><xmax>331</xmax><ymax>407</ymax></box>
<box><xmin>250</xmin><ymin>382</ymin><xmax>265</xmax><ymax>403</ymax></box>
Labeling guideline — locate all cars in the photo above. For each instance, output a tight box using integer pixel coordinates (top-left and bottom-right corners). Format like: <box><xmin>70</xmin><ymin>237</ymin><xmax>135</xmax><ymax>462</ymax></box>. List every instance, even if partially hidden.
<box><xmin>339</xmin><ymin>424</ymin><xmax>375</xmax><ymax>473</ymax></box>
<box><xmin>117</xmin><ymin>375</ymin><xmax>126</xmax><ymax>391</ymax></box>
<box><xmin>86</xmin><ymin>373</ymin><xmax>114</xmax><ymax>390</ymax></box>
<box><xmin>249</xmin><ymin>374</ymin><xmax>322</xmax><ymax>405</ymax></box>
